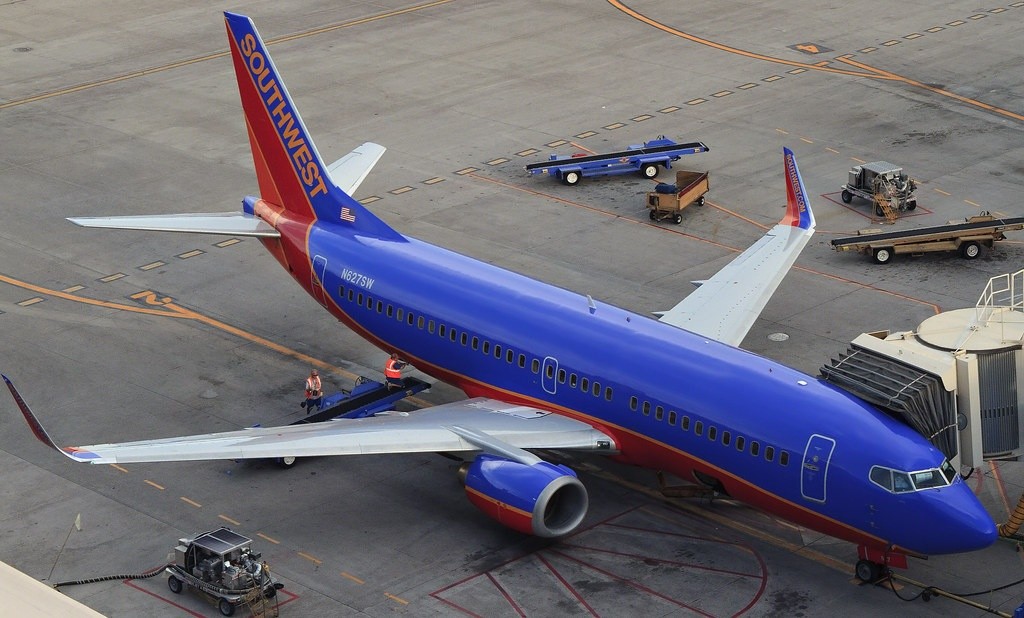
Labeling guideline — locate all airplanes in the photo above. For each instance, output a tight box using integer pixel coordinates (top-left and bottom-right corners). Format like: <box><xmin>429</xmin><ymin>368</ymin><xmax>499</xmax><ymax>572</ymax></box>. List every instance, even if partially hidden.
<box><xmin>0</xmin><ymin>7</ymin><xmax>1007</xmax><ymax>589</ymax></box>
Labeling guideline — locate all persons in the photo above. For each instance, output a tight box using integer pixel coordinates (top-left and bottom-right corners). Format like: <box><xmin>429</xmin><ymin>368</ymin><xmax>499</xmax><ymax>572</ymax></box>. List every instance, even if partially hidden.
<box><xmin>305</xmin><ymin>369</ymin><xmax>324</xmax><ymax>414</ymax></box>
<box><xmin>383</xmin><ymin>353</ymin><xmax>409</xmax><ymax>391</ymax></box>
